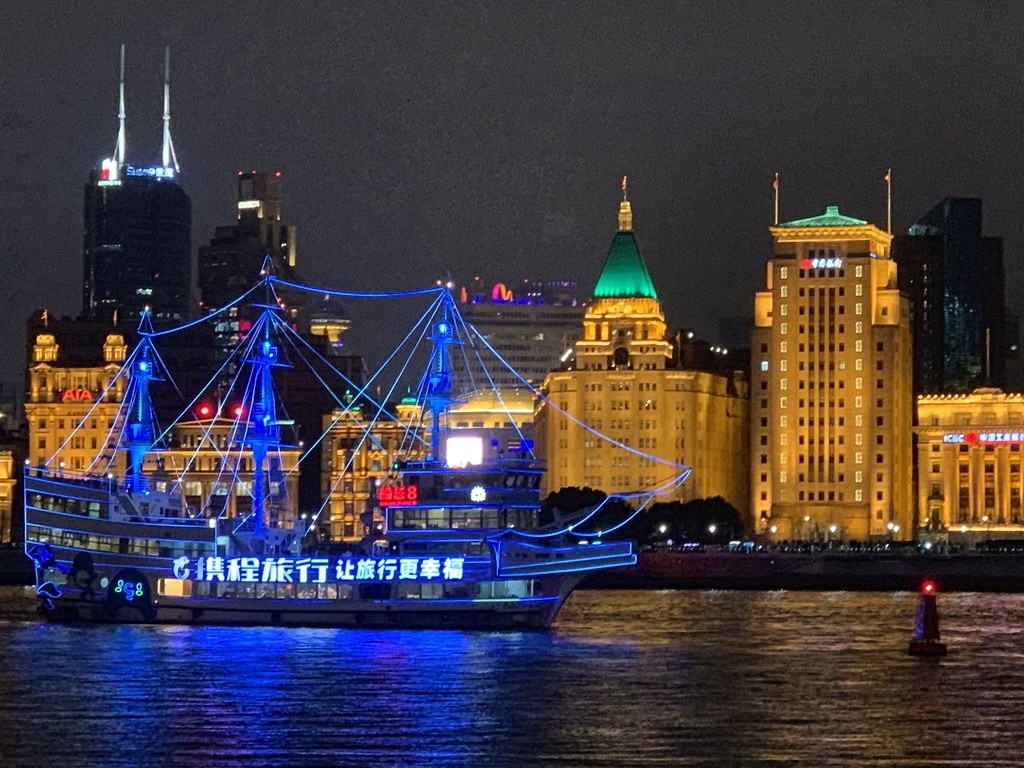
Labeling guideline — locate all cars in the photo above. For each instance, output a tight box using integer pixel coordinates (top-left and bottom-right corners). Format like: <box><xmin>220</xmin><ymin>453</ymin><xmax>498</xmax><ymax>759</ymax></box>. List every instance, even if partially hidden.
<box><xmin>635</xmin><ymin>538</ymin><xmax>1024</xmax><ymax>554</ymax></box>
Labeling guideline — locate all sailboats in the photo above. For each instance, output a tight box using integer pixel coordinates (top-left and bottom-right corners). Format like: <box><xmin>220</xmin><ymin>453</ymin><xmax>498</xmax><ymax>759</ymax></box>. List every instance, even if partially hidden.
<box><xmin>907</xmin><ymin>581</ymin><xmax>949</xmax><ymax>660</ymax></box>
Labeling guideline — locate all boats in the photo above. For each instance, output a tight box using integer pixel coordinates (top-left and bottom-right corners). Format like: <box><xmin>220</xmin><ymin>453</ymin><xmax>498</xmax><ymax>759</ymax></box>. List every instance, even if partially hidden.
<box><xmin>19</xmin><ymin>255</ymin><xmax>698</xmax><ymax>634</ymax></box>
